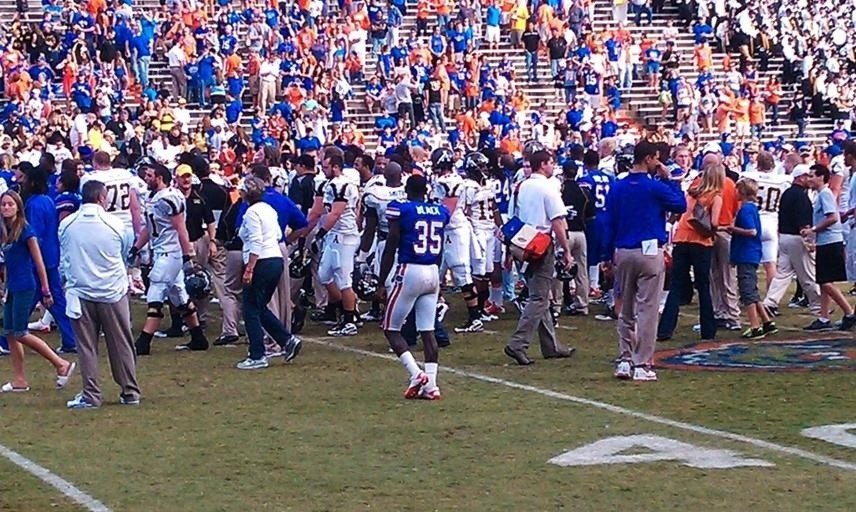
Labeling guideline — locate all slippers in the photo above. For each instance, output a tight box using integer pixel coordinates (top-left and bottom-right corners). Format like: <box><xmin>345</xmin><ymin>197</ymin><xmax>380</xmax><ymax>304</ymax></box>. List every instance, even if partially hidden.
<box><xmin>56</xmin><ymin>361</ymin><xmax>77</xmax><ymax>390</ymax></box>
<box><xmin>0</xmin><ymin>382</ymin><xmax>30</xmax><ymax>393</ymax></box>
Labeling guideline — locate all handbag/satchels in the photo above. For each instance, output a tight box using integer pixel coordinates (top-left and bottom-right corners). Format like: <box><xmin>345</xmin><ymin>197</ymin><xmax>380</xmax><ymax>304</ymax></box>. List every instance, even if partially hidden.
<box><xmin>686</xmin><ymin>190</ymin><xmax>720</xmax><ymax>241</ymax></box>
<box><xmin>494</xmin><ymin>178</ymin><xmax>556</xmax><ymax>264</ymax></box>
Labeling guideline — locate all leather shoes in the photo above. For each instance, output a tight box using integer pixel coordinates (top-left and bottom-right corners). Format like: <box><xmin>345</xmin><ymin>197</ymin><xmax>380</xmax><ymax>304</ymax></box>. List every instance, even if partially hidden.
<box><xmin>553</xmin><ymin>346</ymin><xmax>577</xmax><ymax>358</ymax></box>
<box><xmin>504</xmin><ymin>344</ymin><xmax>531</xmax><ymax>366</ymax></box>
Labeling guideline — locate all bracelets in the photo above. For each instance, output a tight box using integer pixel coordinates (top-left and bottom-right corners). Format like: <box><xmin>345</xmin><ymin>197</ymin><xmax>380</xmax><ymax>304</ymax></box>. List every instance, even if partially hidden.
<box><xmin>245</xmin><ymin>269</ymin><xmax>253</xmax><ymax>276</ymax></box>
<box><xmin>42</xmin><ymin>288</ymin><xmax>51</xmax><ymax>294</ymax></box>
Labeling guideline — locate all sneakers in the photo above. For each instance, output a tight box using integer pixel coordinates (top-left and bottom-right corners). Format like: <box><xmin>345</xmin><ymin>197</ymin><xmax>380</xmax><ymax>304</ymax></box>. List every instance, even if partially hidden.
<box><xmin>741</xmin><ymin>326</ymin><xmax>766</xmax><ymax>341</ymax></box>
<box><xmin>763</xmin><ymin>305</ymin><xmax>781</xmax><ymax>317</ymax></box>
<box><xmin>119</xmin><ymin>391</ymin><xmax>143</xmax><ymax>405</ymax></box>
<box><xmin>155</xmin><ymin>327</ymin><xmax>184</xmax><ymax>340</ymax></box>
<box><xmin>832</xmin><ymin>311</ymin><xmax>856</xmax><ymax>331</ymax></box>
<box><xmin>264</xmin><ymin>344</ymin><xmax>282</xmax><ymax>357</ymax></box>
<box><xmin>387</xmin><ymin>347</ymin><xmax>395</xmax><ymax>354</ymax></box>
<box><xmin>283</xmin><ymin>336</ymin><xmax>302</xmax><ymax>362</ymax></box>
<box><xmin>632</xmin><ymin>367</ymin><xmax>658</xmax><ymax>382</ymax></box>
<box><xmin>174</xmin><ymin>339</ymin><xmax>208</xmax><ymax>354</ymax></box>
<box><xmin>359</xmin><ymin>310</ymin><xmax>383</xmax><ymax>321</ymax></box>
<box><xmin>180</xmin><ymin>319</ymin><xmax>208</xmax><ymax>332</ymax></box>
<box><xmin>310</xmin><ymin>310</ymin><xmax>339</xmax><ymax>325</ymax></box>
<box><xmin>725</xmin><ymin>321</ymin><xmax>742</xmax><ymax>332</ymax></box>
<box><xmin>417</xmin><ymin>385</ymin><xmax>442</xmax><ymax>401</ymax></box>
<box><xmin>435</xmin><ymin>337</ymin><xmax>452</xmax><ymax>349</ymax></box>
<box><xmin>596</xmin><ymin>311</ymin><xmax>618</xmax><ymax>322</ymax></box>
<box><xmin>787</xmin><ymin>293</ymin><xmax>810</xmax><ymax>308</ymax></box>
<box><xmin>763</xmin><ymin>321</ymin><xmax>778</xmax><ymax>336</ymax></box>
<box><xmin>478</xmin><ymin>308</ymin><xmax>500</xmax><ymax>322</ymax></box>
<box><xmin>513</xmin><ymin>294</ymin><xmax>525</xmax><ymax>312</ymax></box>
<box><xmin>57</xmin><ymin>345</ymin><xmax>79</xmax><ymax>354</ymax></box>
<box><xmin>0</xmin><ymin>335</ymin><xmax>11</xmax><ymax>354</ymax></box>
<box><xmin>484</xmin><ymin>299</ymin><xmax>506</xmax><ymax>314</ymax></box>
<box><xmin>453</xmin><ymin>317</ymin><xmax>485</xmax><ymax>333</ymax></box>
<box><xmin>402</xmin><ymin>372</ymin><xmax>430</xmax><ymax>400</ymax></box>
<box><xmin>133</xmin><ymin>342</ymin><xmax>150</xmax><ymax>357</ymax></box>
<box><xmin>800</xmin><ymin>316</ymin><xmax>834</xmax><ymax>332</ymax></box>
<box><xmin>405</xmin><ymin>336</ymin><xmax>419</xmax><ymax>346</ymax></box>
<box><xmin>435</xmin><ymin>300</ymin><xmax>451</xmax><ymax>323</ymax></box>
<box><xmin>693</xmin><ymin>320</ymin><xmax>702</xmax><ymax>332</ymax></box>
<box><xmin>326</xmin><ymin>319</ymin><xmax>359</xmax><ymax>337</ymax></box>
<box><xmin>66</xmin><ymin>394</ymin><xmax>101</xmax><ymax>409</ymax></box>
<box><xmin>214</xmin><ymin>333</ymin><xmax>238</xmax><ymax>345</ymax></box>
<box><xmin>27</xmin><ymin>319</ymin><xmax>51</xmax><ymax>333</ymax></box>
<box><xmin>235</xmin><ymin>355</ymin><xmax>269</xmax><ymax>370</ymax></box>
<box><xmin>562</xmin><ymin>304</ymin><xmax>591</xmax><ymax>316</ymax></box>
<box><xmin>613</xmin><ymin>362</ymin><xmax>631</xmax><ymax>378</ymax></box>
<box><xmin>810</xmin><ymin>304</ymin><xmax>835</xmax><ymax>317</ymax></box>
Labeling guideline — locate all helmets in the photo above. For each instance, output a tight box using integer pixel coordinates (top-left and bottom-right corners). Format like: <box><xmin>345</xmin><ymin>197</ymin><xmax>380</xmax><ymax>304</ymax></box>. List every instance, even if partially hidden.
<box><xmin>290</xmin><ymin>248</ymin><xmax>301</xmax><ymax>259</ymax></box>
<box><xmin>288</xmin><ymin>257</ymin><xmax>312</xmax><ymax>277</ymax></box>
<box><xmin>353</xmin><ymin>270</ymin><xmax>380</xmax><ymax>301</ymax></box>
<box><xmin>463</xmin><ymin>152</ymin><xmax>490</xmax><ymax>186</ymax></box>
<box><xmin>185</xmin><ymin>266</ymin><xmax>212</xmax><ymax>298</ymax></box>
<box><xmin>523</xmin><ymin>139</ymin><xmax>544</xmax><ymax>157</ymax></box>
<box><xmin>431</xmin><ymin>147</ymin><xmax>455</xmax><ymax>171</ymax></box>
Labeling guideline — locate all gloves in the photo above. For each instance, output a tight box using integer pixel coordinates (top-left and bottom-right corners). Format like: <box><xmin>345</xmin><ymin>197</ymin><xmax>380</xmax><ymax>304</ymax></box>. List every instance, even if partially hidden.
<box><xmin>126</xmin><ymin>246</ymin><xmax>141</xmax><ymax>268</ymax></box>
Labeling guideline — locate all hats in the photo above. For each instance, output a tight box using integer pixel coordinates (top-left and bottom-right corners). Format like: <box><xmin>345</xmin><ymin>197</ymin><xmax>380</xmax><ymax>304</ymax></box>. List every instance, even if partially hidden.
<box><xmin>838</xmin><ymin>119</ymin><xmax>844</xmax><ymax>124</ymax></box>
<box><xmin>175</xmin><ymin>163</ymin><xmax>193</xmax><ymax>177</ymax></box>
<box><xmin>11</xmin><ymin>161</ymin><xmax>34</xmax><ymax>169</ymax></box>
<box><xmin>823</xmin><ymin>145</ymin><xmax>841</xmax><ymax>155</ymax></box>
<box><xmin>799</xmin><ymin>145</ymin><xmax>811</xmax><ymax>156</ymax></box>
<box><xmin>792</xmin><ymin>164</ymin><xmax>810</xmax><ymax>177</ymax></box>
<box><xmin>745</xmin><ymin>142</ymin><xmax>760</xmax><ymax>153</ymax></box>
<box><xmin>702</xmin><ymin>142</ymin><xmax>722</xmax><ymax>155</ymax></box>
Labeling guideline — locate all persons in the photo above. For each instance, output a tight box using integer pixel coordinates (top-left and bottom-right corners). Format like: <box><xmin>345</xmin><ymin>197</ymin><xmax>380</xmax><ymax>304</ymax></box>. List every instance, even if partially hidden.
<box><xmin>847</xmin><ymin>286</ymin><xmax>856</xmax><ymax>295</ymax></box>
<box><xmin>56</xmin><ymin>179</ymin><xmax>143</xmax><ymax>411</ymax></box>
<box><xmin>1</xmin><ymin>153</ymin><xmax>18</xmax><ymax>190</ymax></box>
<box><xmin>54</xmin><ymin>169</ymin><xmax>84</xmax><ymax>222</ymax></box>
<box><xmin>211</xmin><ymin>196</ymin><xmax>245</xmax><ymax>345</ymax></box>
<box><xmin>655</xmin><ymin>160</ymin><xmax>726</xmax><ymax>342</ymax></box>
<box><xmin>745</xmin><ymin>151</ymin><xmax>792</xmax><ymax>312</ymax></box>
<box><xmin>482</xmin><ymin>148</ymin><xmax>509</xmax><ymax>317</ymax></box>
<box><xmin>503</xmin><ymin>150</ymin><xmax>576</xmax><ymax>368</ymax></box>
<box><xmin>802</xmin><ymin>164</ymin><xmax>855</xmax><ymax>332</ymax></box>
<box><xmin>190</xmin><ymin>157</ymin><xmax>231</xmax><ymax>325</ymax></box>
<box><xmin>377</xmin><ymin>174</ymin><xmax>451</xmax><ymax>400</ymax></box>
<box><xmin>127</xmin><ymin>154</ymin><xmax>155</xmax><ymax>303</ymax></box>
<box><xmin>387</xmin><ymin>303</ymin><xmax>451</xmax><ymax>353</ymax></box>
<box><xmin>34</xmin><ymin>151</ymin><xmax>57</xmax><ymax>195</ymax></box>
<box><xmin>516</xmin><ymin>158</ymin><xmax>559</xmax><ymax>328</ymax></box>
<box><xmin>605</xmin><ymin>139</ymin><xmax>687</xmax><ymax>383</ymax></box>
<box><xmin>699</xmin><ymin>154</ymin><xmax>744</xmax><ymax>332</ymax></box>
<box><xmin>210</xmin><ymin>159</ymin><xmax>245</xmax><ymax>187</ymax></box>
<box><xmin>552</xmin><ymin>158</ymin><xmax>591</xmax><ymax>317</ymax></box>
<box><xmin>1</xmin><ymin>190</ymin><xmax>77</xmax><ymax>394</ymax></box>
<box><xmin>761</xmin><ymin>163</ymin><xmax>835</xmax><ymax>319</ymax></box>
<box><xmin>1</xmin><ymin>169</ymin><xmax>77</xmax><ymax>355</ymax></box>
<box><xmin>74</xmin><ymin>159</ymin><xmax>85</xmax><ymax>177</ymax></box>
<box><xmin>838</xmin><ymin>140</ymin><xmax>856</xmax><ymax>283</ymax></box>
<box><xmin>143</xmin><ymin>164</ymin><xmax>155</xmax><ymax>202</ymax></box>
<box><xmin>234</xmin><ymin>165</ymin><xmax>309</xmax><ymax>358</ymax></box>
<box><xmin>462</xmin><ymin>152</ymin><xmax>505</xmax><ymax>321</ymax></box>
<box><xmin>10</xmin><ymin>160</ymin><xmax>31</xmax><ymax>197</ymax></box>
<box><xmin>173</xmin><ymin>162</ymin><xmax>217</xmax><ymax>339</ymax></box>
<box><xmin>579</xmin><ymin>148</ymin><xmax>613</xmax><ymax>299</ymax></box>
<box><xmin>359</xmin><ymin>161</ymin><xmax>407</xmax><ymax>305</ymax></box>
<box><xmin>134</xmin><ymin>163</ymin><xmax>210</xmax><ymax>357</ymax></box>
<box><xmin>725</xmin><ymin>177</ymin><xmax>778</xmax><ymax>340</ymax></box>
<box><xmin>61</xmin><ymin>158</ymin><xmax>77</xmax><ymax>175</ymax></box>
<box><xmin>79</xmin><ymin>150</ymin><xmax>135</xmax><ymax>339</ymax></box>
<box><xmin>311</xmin><ymin>145</ymin><xmax>365</xmax><ymax>337</ymax></box>
<box><xmin>0</xmin><ymin>2</ymin><xmax>844</xmax><ymax>162</ymax></box>
<box><xmin>287</xmin><ymin>154</ymin><xmax>317</xmax><ymax>301</ymax></box>
<box><xmin>236</xmin><ymin>172</ymin><xmax>301</xmax><ymax>370</ymax></box>
<box><xmin>421</xmin><ymin>147</ymin><xmax>485</xmax><ymax>334</ymax></box>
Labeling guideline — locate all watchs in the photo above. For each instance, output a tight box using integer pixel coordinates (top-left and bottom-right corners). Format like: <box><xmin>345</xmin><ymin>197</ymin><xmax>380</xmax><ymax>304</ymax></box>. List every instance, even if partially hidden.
<box><xmin>209</xmin><ymin>236</ymin><xmax>220</xmax><ymax>244</ymax></box>
<box><xmin>43</xmin><ymin>294</ymin><xmax>52</xmax><ymax>298</ymax></box>
<box><xmin>810</xmin><ymin>224</ymin><xmax>818</xmax><ymax>233</ymax></box>
